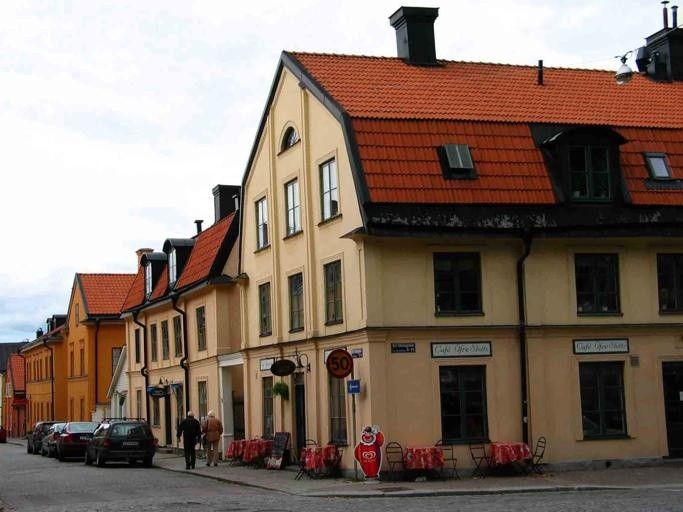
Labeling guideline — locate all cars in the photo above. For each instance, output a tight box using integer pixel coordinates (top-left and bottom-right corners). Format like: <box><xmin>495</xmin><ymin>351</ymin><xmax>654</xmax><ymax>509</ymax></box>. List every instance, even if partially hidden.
<box><xmin>51</xmin><ymin>421</ymin><xmax>100</xmax><ymax>461</ymax></box>
<box><xmin>39</xmin><ymin>422</ymin><xmax>66</xmax><ymax>457</ymax></box>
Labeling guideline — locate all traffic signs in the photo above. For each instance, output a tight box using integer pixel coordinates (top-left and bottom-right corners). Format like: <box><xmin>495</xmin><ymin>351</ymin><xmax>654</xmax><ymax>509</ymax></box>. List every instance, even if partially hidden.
<box><xmin>324</xmin><ymin>348</ymin><xmax>355</xmax><ymax>379</ymax></box>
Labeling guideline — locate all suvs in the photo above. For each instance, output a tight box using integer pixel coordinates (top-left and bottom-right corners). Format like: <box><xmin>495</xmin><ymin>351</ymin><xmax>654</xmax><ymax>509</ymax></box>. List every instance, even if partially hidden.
<box><xmin>82</xmin><ymin>416</ymin><xmax>158</xmax><ymax>467</ymax></box>
<box><xmin>25</xmin><ymin>421</ymin><xmax>64</xmax><ymax>455</ymax></box>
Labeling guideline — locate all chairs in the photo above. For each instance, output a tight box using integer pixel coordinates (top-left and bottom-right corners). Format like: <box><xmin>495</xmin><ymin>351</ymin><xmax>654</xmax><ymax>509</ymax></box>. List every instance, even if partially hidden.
<box><xmin>386</xmin><ymin>441</ymin><xmax>415</xmax><ymax>482</ymax></box>
<box><xmin>290</xmin><ymin>439</ymin><xmax>344</xmax><ymax>480</ymax></box>
<box><xmin>523</xmin><ymin>436</ymin><xmax>546</xmax><ymax>474</ymax></box>
<box><xmin>468</xmin><ymin>439</ymin><xmax>493</xmax><ymax>478</ymax></box>
<box><xmin>435</xmin><ymin>440</ymin><xmax>460</xmax><ymax>481</ymax></box>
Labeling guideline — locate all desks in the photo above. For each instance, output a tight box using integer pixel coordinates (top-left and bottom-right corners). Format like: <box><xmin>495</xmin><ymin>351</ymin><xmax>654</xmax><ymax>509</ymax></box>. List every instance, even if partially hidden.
<box><xmin>402</xmin><ymin>446</ymin><xmax>446</xmax><ymax>481</ymax></box>
<box><xmin>224</xmin><ymin>438</ymin><xmax>274</xmax><ymax>470</ymax></box>
<box><xmin>487</xmin><ymin>442</ymin><xmax>533</xmax><ymax>476</ymax></box>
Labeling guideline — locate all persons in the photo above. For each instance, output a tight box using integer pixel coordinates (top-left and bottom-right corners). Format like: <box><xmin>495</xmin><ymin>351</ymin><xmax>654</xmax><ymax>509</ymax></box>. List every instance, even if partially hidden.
<box><xmin>176</xmin><ymin>410</ymin><xmax>223</xmax><ymax>470</ymax></box>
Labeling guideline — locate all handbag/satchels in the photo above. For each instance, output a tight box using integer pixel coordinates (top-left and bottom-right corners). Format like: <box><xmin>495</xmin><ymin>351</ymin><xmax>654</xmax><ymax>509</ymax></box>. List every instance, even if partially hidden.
<box><xmin>201</xmin><ymin>433</ymin><xmax>206</xmax><ymax>448</ymax></box>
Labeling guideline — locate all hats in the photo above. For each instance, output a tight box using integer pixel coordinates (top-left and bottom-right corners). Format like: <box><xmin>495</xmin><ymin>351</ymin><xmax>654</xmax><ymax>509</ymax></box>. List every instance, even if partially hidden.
<box><xmin>188</xmin><ymin>411</ymin><xmax>195</xmax><ymax>416</ymax></box>
<box><xmin>208</xmin><ymin>410</ymin><xmax>215</xmax><ymax>416</ymax></box>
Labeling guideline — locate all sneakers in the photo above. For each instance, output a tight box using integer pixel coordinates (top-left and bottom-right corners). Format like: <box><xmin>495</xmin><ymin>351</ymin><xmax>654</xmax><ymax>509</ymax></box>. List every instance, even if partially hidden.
<box><xmin>207</xmin><ymin>464</ymin><xmax>218</xmax><ymax>467</ymax></box>
<box><xmin>186</xmin><ymin>466</ymin><xmax>195</xmax><ymax>470</ymax></box>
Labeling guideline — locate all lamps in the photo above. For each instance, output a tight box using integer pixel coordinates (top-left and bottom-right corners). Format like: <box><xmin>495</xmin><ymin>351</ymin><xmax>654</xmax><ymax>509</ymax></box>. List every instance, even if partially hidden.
<box><xmin>158</xmin><ymin>376</ymin><xmax>168</xmax><ymax>388</ymax></box>
<box><xmin>296</xmin><ymin>353</ymin><xmax>310</xmax><ymax>373</ymax></box>
<box><xmin>612</xmin><ymin>48</ymin><xmax>642</xmax><ymax>85</ymax></box>
